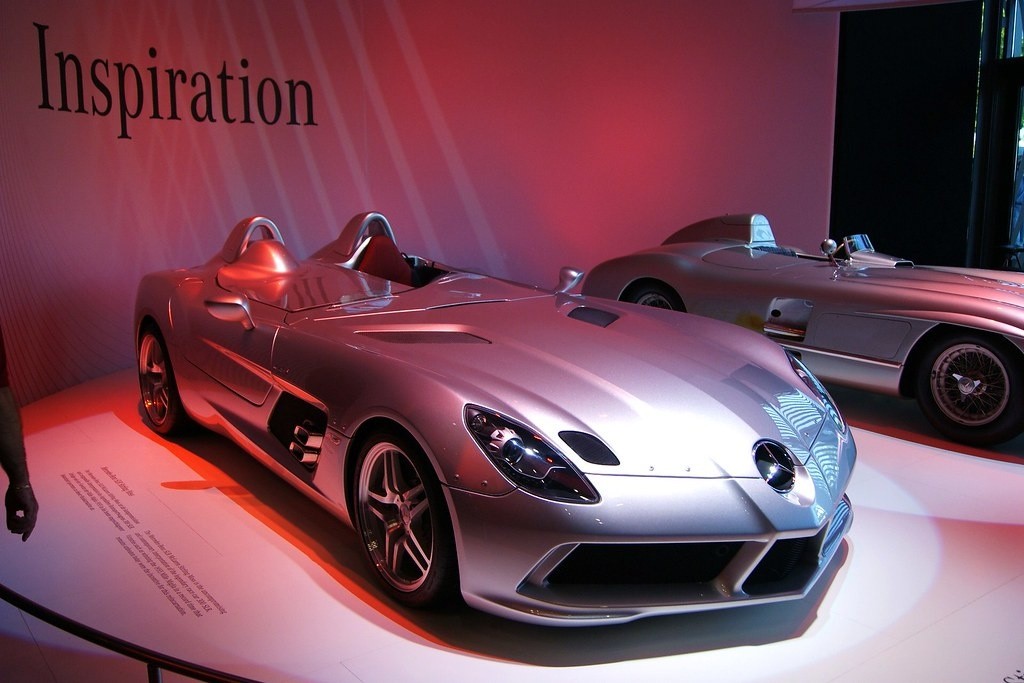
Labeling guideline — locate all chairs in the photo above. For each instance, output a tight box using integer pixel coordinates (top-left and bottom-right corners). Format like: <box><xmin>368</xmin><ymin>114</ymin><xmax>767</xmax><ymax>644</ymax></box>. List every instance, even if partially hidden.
<box><xmin>350</xmin><ymin>234</ymin><xmax>412</xmax><ymax>287</ymax></box>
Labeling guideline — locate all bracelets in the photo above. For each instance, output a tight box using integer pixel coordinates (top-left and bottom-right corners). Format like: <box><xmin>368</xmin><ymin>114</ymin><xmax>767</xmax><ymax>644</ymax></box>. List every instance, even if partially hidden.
<box><xmin>11</xmin><ymin>485</ymin><xmax>31</xmax><ymax>491</ymax></box>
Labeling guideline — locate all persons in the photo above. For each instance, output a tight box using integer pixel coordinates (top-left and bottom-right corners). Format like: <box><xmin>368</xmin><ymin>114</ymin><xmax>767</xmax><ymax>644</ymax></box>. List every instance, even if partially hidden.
<box><xmin>0</xmin><ymin>329</ymin><xmax>39</xmax><ymax>541</ymax></box>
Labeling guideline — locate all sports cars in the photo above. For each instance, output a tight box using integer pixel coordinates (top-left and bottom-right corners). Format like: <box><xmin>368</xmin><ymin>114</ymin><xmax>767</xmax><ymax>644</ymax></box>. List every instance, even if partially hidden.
<box><xmin>128</xmin><ymin>210</ymin><xmax>857</xmax><ymax>631</ymax></box>
<box><xmin>581</xmin><ymin>211</ymin><xmax>1024</xmax><ymax>450</ymax></box>
